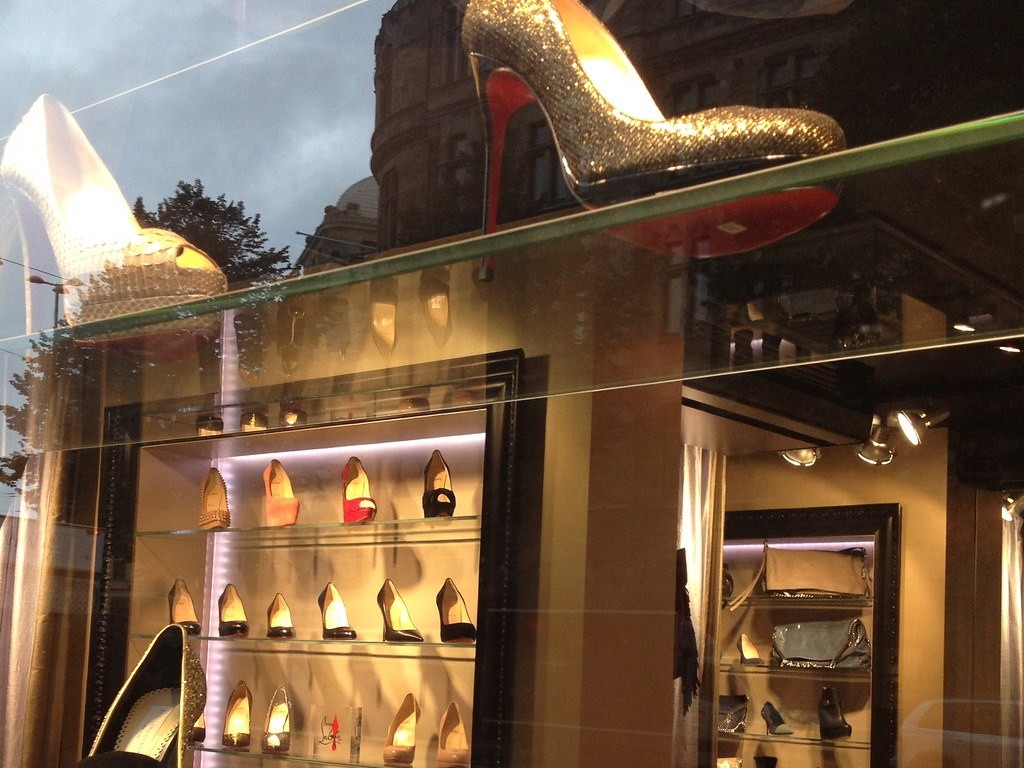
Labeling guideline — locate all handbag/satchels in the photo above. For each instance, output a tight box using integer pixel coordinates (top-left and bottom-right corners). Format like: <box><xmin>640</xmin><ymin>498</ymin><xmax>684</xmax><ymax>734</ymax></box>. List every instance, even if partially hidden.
<box><xmin>718</xmin><ymin>693</ymin><xmax>749</xmax><ymax>732</ymax></box>
<box><xmin>771</xmin><ymin>610</ymin><xmax>871</xmax><ymax>671</ymax></box>
<box><xmin>728</xmin><ymin>540</ymin><xmax>866</xmax><ymax>612</ymax></box>
<box><xmin>717</xmin><ymin>757</ymin><xmax>743</xmax><ymax>768</ymax></box>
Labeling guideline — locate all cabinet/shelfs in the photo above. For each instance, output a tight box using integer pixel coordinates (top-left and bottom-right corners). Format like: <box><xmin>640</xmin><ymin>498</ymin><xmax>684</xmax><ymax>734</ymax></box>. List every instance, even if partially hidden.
<box><xmin>85</xmin><ymin>348</ymin><xmax>902</xmax><ymax>768</ymax></box>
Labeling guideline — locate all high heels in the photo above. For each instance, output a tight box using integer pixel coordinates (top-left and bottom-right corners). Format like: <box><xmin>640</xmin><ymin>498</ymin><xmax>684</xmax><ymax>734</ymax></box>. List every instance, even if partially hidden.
<box><xmin>761</xmin><ymin>701</ymin><xmax>794</xmax><ymax>734</ymax></box>
<box><xmin>818</xmin><ymin>686</ymin><xmax>852</xmax><ymax>740</ymax></box>
<box><xmin>0</xmin><ymin>93</ymin><xmax>227</xmax><ymax>341</ymax></box>
<box><xmin>460</xmin><ymin>0</ymin><xmax>847</xmax><ymax>258</ymax></box>
<box><xmin>90</xmin><ymin>623</ymin><xmax>206</xmax><ymax>768</ymax></box>
<box><xmin>737</xmin><ymin>633</ymin><xmax>765</xmax><ymax>666</ymax></box>
<box><xmin>164</xmin><ymin>356</ymin><xmax>475</xmax><ymax>768</ymax></box>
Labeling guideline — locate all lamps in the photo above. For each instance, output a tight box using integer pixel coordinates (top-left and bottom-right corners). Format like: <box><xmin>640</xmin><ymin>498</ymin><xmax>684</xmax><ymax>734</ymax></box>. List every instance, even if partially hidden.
<box><xmin>779</xmin><ymin>447</ymin><xmax>824</xmax><ymax>468</ymax></box>
<box><xmin>896</xmin><ymin>407</ymin><xmax>952</xmax><ymax>445</ymax></box>
<box><xmin>859</xmin><ymin>410</ymin><xmax>901</xmax><ymax>465</ymax></box>
